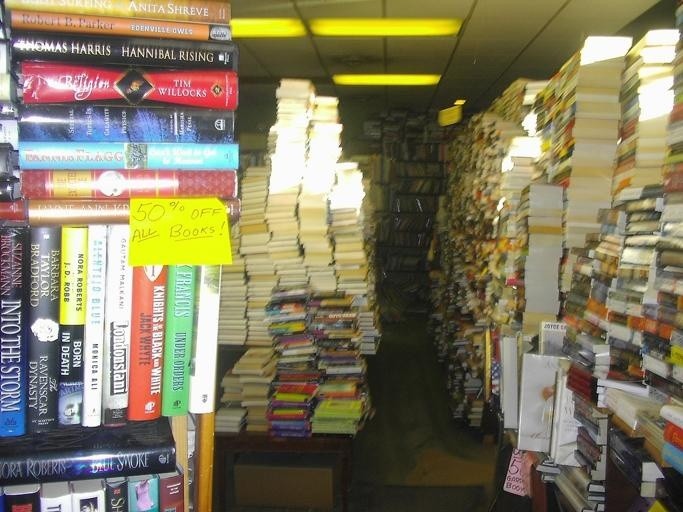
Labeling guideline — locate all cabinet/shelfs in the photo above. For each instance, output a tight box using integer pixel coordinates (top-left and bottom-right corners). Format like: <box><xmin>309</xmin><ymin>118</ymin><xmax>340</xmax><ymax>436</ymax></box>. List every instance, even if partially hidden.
<box><xmin>0</xmin><ymin>198</ymin><xmax>243</xmax><ymax>512</ymax></box>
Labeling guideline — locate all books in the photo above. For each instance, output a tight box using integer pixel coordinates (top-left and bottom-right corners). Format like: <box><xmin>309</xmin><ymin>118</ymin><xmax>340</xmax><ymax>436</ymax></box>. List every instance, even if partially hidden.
<box><xmin>0</xmin><ymin>1</ymin><xmax>682</xmax><ymax>511</ymax></box>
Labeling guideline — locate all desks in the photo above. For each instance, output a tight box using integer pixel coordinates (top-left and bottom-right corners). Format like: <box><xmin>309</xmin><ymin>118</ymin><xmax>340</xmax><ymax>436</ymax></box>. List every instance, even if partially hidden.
<box><xmin>217</xmin><ymin>436</ymin><xmax>350</xmax><ymax>511</ymax></box>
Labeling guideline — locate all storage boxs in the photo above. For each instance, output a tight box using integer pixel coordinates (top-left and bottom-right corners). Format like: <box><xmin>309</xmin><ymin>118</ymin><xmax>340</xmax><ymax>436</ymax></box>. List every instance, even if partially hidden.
<box><xmin>235</xmin><ymin>454</ymin><xmax>336</xmax><ymax>511</ymax></box>
